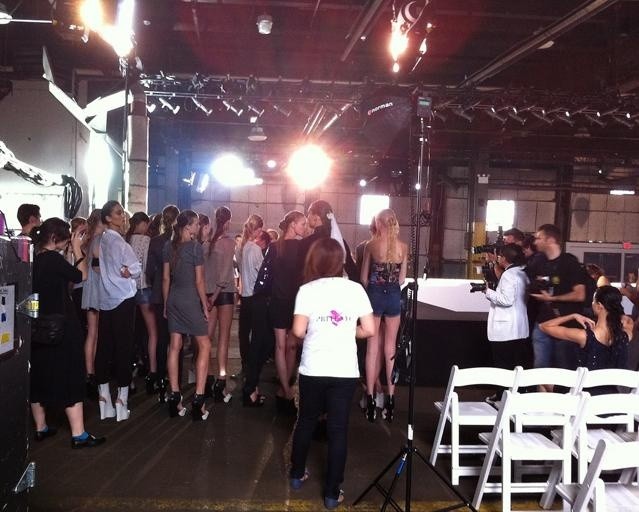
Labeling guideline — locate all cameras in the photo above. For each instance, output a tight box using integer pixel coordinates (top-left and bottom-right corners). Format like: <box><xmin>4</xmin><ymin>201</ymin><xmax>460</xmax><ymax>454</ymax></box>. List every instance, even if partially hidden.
<box><xmin>469</xmin><ymin>261</ymin><xmax>498</xmax><ymax>293</ymax></box>
<box><xmin>472</xmin><ymin>226</ymin><xmax>505</xmax><ymax>257</ymax></box>
<box><xmin>70</xmin><ymin>231</ymin><xmax>89</xmax><ymax>243</ymax></box>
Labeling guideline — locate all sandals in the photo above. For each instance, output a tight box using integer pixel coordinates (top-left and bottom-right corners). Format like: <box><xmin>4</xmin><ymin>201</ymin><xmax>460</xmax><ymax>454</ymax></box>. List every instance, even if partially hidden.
<box><xmin>324</xmin><ymin>488</ymin><xmax>345</xmax><ymax>509</ymax></box>
<box><xmin>291</xmin><ymin>468</ymin><xmax>309</xmax><ymax>489</ymax></box>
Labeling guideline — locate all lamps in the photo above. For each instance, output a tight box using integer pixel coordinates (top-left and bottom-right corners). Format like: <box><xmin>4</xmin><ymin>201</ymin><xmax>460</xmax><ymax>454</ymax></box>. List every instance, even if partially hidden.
<box><xmin>253</xmin><ymin>7</ymin><xmax>276</xmax><ymax>37</ymax></box>
<box><xmin>434</xmin><ymin>99</ymin><xmax>638</xmax><ymax>126</ymax></box>
<box><xmin>245</xmin><ymin>118</ymin><xmax>267</xmax><ymax>145</ymax></box>
<box><xmin>142</xmin><ymin>92</ymin><xmax>344</xmax><ymax>121</ymax></box>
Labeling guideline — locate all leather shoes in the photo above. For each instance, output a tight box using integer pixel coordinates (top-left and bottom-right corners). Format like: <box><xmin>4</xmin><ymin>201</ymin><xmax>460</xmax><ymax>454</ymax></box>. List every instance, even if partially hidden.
<box><xmin>72</xmin><ymin>433</ymin><xmax>106</xmax><ymax>449</ymax></box>
<box><xmin>36</xmin><ymin>423</ymin><xmax>58</xmax><ymax>442</ymax></box>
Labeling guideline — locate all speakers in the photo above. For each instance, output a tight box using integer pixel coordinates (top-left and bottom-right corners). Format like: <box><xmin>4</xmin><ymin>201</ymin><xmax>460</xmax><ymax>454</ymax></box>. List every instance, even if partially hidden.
<box><xmin>359</xmin><ymin>95</ymin><xmax>411</xmax><ymax>152</ymax></box>
<box><xmin>0</xmin><ymin>236</ymin><xmax>34</xmax><ymax>512</ymax></box>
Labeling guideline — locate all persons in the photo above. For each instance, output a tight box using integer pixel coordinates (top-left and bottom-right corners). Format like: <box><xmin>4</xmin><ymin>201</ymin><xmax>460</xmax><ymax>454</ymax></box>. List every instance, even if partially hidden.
<box><xmin>18</xmin><ymin>199</ymin><xmax>314</xmax><ymax>449</ymax></box>
<box><xmin>485</xmin><ymin>224</ymin><xmax>639</xmax><ymax>432</ymax></box>
<box><xmin>287</xmin><ymin>201</ymin><xmax>407</xmax><ymax>423</ymax></box>
<box><xmin>288</xmin><ymin>238</ymin><xmax>376</xmax><ymax>507</ymax></box>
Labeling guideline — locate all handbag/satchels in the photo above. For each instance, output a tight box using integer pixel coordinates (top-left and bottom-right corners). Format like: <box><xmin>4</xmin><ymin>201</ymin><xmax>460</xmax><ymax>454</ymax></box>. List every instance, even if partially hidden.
<box><xmin>31</xmin><ymin>318</ymin><xmax>57</xmax><ymax>345</ymax></box>
<box><xmin>392</xmin><ymin>337</ymin><xmax>416</xmax><ymax>386</ymax></box>
<box><xmin>253</xmin><ymin>243</ymin><xmax>273</xmax><ymax>296</ymax></box>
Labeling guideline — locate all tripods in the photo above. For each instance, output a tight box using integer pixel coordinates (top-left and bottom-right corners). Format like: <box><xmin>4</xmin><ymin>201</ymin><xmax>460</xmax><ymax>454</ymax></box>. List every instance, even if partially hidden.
<box><xmin>352</xmin><ymin>120</ymin><xmax>478</xmax><ymax>512</ymax></box>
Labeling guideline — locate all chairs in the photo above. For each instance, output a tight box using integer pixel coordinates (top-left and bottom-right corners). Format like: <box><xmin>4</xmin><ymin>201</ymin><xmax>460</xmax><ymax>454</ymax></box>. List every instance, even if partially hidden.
<box><xmin>429</xmin><ymin>359</ymin><xmax>639</xmax><ymax>512</ymax></box>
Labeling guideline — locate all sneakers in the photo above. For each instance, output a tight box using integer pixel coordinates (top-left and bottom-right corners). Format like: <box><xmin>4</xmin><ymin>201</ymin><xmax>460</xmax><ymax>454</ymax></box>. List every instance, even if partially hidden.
<box><xmin>486</xmin><ymin>394</ymin><xmax>498</xmax><ymax>404</ymax></box>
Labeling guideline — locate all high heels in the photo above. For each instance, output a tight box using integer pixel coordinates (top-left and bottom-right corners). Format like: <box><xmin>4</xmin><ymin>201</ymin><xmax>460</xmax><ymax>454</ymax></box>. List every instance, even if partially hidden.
<box><xmin>381</xmin><ymin>394</ymin><xmax>395</xmax><ymax>422</ymax></box>
<box><xmin>256</xmin><ymin>393</ymin><xmax>268</xmax><ymax>400</ymax></box>
<box><xmin>115</xmin><ymin>387</ymin><xmax>131</xmax><ymax>421</ymax></box>
<box><xmin>242</xmin><ymin>387</ymin><xmax>264</xmax><ymax>407</ymax></box>
<box><xmin>365</xmin><ymin>395</ymin><xmax>376</xmax><ymax>422</ymax></box>
<box><xmin>97</xmin><ymin>382</ymin><xmax>117</xmax><ymax>421</ymax></box>
<box><xmin>145</xmin><ymin>373</ymin><xmax>233</xmax><ymax>420</ymax></box>
